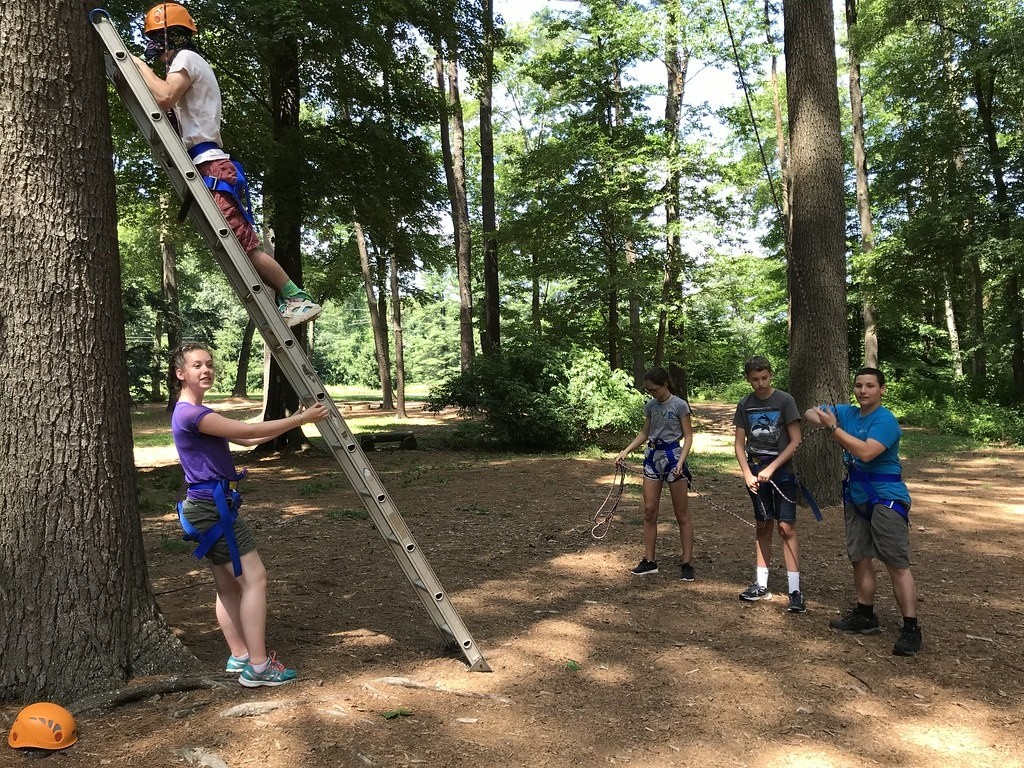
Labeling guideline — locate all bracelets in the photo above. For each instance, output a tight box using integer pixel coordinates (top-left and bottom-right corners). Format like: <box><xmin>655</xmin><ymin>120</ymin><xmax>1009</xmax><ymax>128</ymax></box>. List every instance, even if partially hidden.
<box><xmin>301</xmin><ymin>414</ymin><xmax>303</xmax><ymax>425</ymax></box>
<box><xmin>829</xmin><ymin>424</ymin><xmax>837</xmax><ymax>434</ymax></box>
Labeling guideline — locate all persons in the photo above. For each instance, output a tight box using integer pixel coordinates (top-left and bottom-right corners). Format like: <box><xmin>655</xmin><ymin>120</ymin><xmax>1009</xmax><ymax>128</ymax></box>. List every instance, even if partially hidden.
<box><xmin>171</xmin><ymin>343</ymin><xmax>329</xmax><ymax>687</ymax></box>
<box><xmin>733</xmin><ymin>356</ymin><xmax>806</xmax><ymax>612</ymax></box>
<box><xmin>617</xmin><ymin>367</ymin><xmax>695</xmax><ymax>581</ymax></box>
<box><xmin>805</xmin><ymin>368</ymin><xmax>923</xmax><ymax>655</ymax></box>
<box><xmin>131</xmin><ymin>3</ymin><xmax>322</xmax><ymax>328</ymax></box>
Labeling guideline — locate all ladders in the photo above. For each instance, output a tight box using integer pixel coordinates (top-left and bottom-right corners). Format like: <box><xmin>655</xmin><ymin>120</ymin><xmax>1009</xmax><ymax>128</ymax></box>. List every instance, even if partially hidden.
<box><xmin>91</xmin><ymin>7</ymin><xmax>490</xmax><ymax>672</ymax></box>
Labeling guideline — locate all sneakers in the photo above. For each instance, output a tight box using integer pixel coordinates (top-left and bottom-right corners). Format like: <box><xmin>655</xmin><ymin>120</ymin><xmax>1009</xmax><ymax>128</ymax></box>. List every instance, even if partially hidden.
<box><xmin>680</xmin><ymin>563</ymin><xmax>695</xmax><ymax>582</ymax></box>
<box><xmin>280</xmin><ymin>299</ymin><xmax>322</xmax><ymax>327</ymax></box>
<box><xmin>226</xmin><ymin>653</ymin><xmax>250</xmax><ymax>673</ymax></box>
<box><xmin>828</xmin><ymin>608</ymin><xmax>881</xmax><ymax>636</ymax></box>
<box><xmin>738</xmin><ymin>581</ymin><xmax>772</xmax><ymax>601</ymax></box>
<box><xmin>892</xmin><ymin>625</ymin><xmax>923</xmax><ymax>656</ymax></box>
<box><xmin>787</xmin><ymin>590</ymin><xmax>806</xmax><ymax>613</ymax></box>
<box><xmin>631</xmin><ymin>557</ymin><xmax>659</xmax><ymax>575</ymax></box>
<box><xmin>238</xmin><ymin>650</ymin><xmax>297</xmax><ymax>688</ymax></box>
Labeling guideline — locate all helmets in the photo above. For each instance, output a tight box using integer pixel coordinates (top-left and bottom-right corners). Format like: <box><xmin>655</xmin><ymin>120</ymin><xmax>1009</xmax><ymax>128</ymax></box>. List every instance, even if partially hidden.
<box><xmin>144</xmin><ymin>3</ymin><xmax>198</xmax><ymax>36</ymax></box>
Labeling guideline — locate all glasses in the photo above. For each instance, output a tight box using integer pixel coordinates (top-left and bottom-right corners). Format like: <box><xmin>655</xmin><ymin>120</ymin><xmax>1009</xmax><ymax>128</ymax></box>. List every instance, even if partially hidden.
<box><xmin>643</xmin><ymin>384</ymin><xmax>666</xmax><ymax>395</ymax></box>
